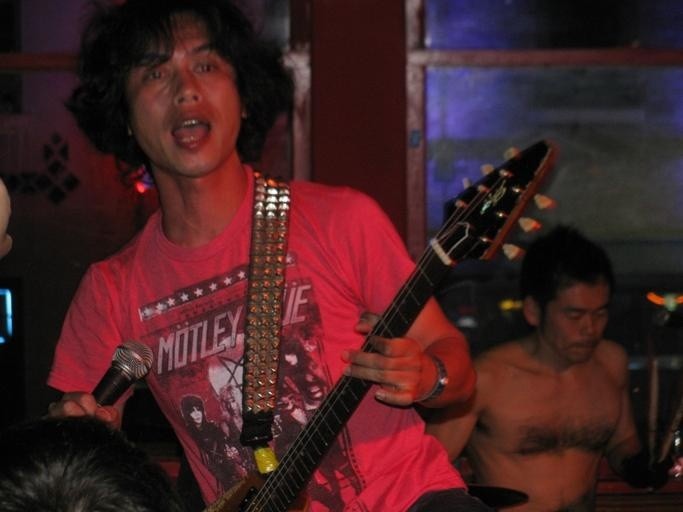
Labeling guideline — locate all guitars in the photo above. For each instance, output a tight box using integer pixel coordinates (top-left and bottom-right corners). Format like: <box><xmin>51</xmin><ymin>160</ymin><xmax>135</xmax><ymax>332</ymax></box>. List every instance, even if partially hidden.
<box><xmin>202</xmin><ymin>138</ymin><xmax>560</xmax><ymax>512</ymax></box>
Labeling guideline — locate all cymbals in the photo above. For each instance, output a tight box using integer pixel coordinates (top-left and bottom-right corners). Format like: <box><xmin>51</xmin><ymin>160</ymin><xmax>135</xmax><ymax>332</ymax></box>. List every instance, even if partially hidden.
<box><xmin>467</xmin><ymin>484</ymin><xmax>528</xmax><ymax>508</ymax></box>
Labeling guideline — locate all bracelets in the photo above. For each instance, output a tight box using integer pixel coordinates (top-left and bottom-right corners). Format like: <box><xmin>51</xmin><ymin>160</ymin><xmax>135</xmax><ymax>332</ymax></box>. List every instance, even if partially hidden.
<box><xmin>416</xmin><ymin>351</ymin><xmax>448</xmax><ymax>406</ymax></box>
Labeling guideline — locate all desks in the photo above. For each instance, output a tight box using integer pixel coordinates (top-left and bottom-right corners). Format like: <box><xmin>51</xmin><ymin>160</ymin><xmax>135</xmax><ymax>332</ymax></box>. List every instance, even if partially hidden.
<box><xmin>625</xmin><ymin>354</ymin><xmax>682</xmax><ymax>460</ymax></box>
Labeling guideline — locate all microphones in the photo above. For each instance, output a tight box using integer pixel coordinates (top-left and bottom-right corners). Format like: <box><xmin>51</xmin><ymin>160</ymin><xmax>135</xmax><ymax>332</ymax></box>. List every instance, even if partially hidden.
<box><xmin>91</xmin><ymin>339</ymin><xmax>153</xmax><ymax>407</ymax></box>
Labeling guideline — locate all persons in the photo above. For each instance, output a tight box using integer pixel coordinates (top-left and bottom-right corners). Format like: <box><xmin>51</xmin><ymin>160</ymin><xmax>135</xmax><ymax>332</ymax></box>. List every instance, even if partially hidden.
<box><xmin>425</xmin><ymin>223</ymin><xmax>683</xmax><ymax>512</ymax></box>
<box><xmin>47</xmin><ymin>0</ymin><xmax>495</xmax><ymax>512</ymax></box>
<box><xmin>1</xmin><ymin>415</ymin><xmax>180</xmax><ymax>511</ymax></box>
